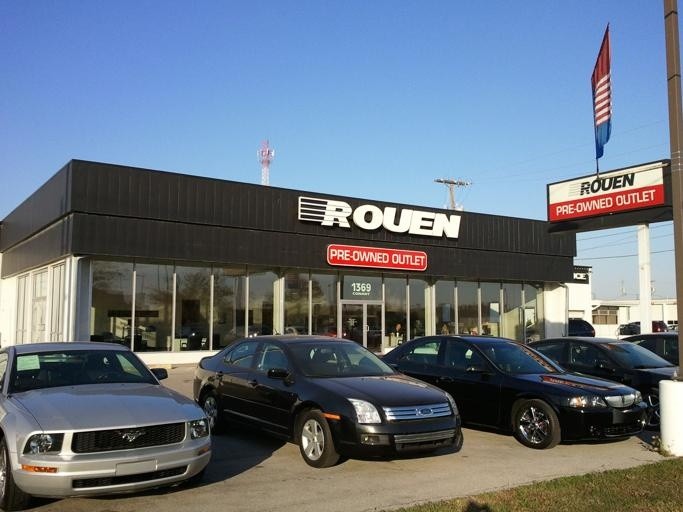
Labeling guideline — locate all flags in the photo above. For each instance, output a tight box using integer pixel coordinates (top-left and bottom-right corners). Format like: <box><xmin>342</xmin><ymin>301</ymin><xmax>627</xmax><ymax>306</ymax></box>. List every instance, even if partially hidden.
<box><xmin>590</xmin><ymin>22</ymin><xmax>614</xmax><ymax>160</ymax></box>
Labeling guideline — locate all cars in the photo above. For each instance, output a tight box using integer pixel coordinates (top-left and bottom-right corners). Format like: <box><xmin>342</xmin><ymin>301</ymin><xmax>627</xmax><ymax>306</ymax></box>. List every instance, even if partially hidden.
<box><xmin>193</xmin><ymin>331</ymin><xmax>461</xmax><ymax>469</ymax></box>
<box><xmin>178</xmin><ymin>315</ymin><xmax>309</xmax><ymax>344</ymax></box>
<box><xmin>0</xmin><ymin>342</ymin><xmax>212</xmax><ymax>512</ymax></box>
<box><xmin>359</xmin><ymin>334</ymin><xmax>649</xmax><ymax>449</ymax></box>
<box><xmin>524</xmin><ymin>319</ymin><xmax>678</xmax><ymax>428</ymax></box>
